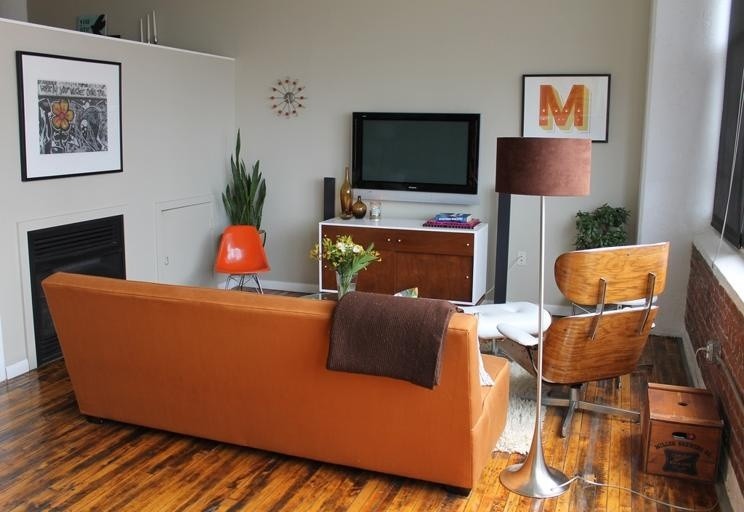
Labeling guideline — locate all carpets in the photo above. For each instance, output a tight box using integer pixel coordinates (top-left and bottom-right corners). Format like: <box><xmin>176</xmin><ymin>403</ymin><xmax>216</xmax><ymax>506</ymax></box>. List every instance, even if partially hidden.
<box><xmin>492</xmin><ymin>360</ymin><xmax>550</xmax><ymax>455</ymax></box>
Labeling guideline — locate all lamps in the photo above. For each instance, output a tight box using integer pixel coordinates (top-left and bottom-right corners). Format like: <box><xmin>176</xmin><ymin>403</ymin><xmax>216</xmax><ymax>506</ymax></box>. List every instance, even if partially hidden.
<box><xmin>495</xmin><ymin>137</ymin><xmax>593</xmax><ymax>499</ymax></box>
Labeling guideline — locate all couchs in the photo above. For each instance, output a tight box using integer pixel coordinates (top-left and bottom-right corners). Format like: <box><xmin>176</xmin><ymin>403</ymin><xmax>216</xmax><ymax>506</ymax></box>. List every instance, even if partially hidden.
<box><xmin>43</xmin><ymin>272</ymin><xmax>510</xmax><ymax>495</ymax></box>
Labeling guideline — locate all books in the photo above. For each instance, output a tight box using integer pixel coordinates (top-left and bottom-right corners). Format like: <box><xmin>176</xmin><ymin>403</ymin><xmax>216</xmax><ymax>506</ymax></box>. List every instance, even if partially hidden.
<box><xmin>423</xmin><ymin>217</ymin><xmax>483</xmax><ymax>229</ymax></box>
<box><xmin>434</xmin><ymin>212</ymin><xmax>473</xmax><ymax>222</ymax></box>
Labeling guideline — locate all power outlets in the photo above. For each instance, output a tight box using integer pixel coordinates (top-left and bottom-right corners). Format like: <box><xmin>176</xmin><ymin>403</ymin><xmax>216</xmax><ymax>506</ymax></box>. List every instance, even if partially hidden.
<box><xmin>706</xmin><ymin>340</ymin><xmax>719</xmax><ymax>364</ymax></box>
<box><xmin>516</xmin><ymin>251</ymin><xmax>527</xmax><ymax>266</ymax></box>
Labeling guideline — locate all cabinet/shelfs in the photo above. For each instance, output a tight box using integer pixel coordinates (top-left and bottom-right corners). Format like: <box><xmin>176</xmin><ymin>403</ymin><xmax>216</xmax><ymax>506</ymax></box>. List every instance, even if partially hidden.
<box><xmin>319</xmin><ymin>223</ymin><xmax>488</xmax><ymax>306</ymax></box>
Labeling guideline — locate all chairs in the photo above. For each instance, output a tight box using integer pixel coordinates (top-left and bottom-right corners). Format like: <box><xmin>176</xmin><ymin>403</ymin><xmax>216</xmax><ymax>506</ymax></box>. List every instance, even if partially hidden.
<box><xmin>496</xmin><ymin>240</ymin><xmax>671</xmax><ymax>440</ymax></box>
<box><xmin>214</xmin><ymin>224</ymin><xmax>271</xmax><ymax>294</ymax></box>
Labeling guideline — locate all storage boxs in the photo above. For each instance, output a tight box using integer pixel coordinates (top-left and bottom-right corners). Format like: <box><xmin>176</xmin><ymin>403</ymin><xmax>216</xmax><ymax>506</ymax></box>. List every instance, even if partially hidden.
<box><xmin>641</xmin><ymin>383</ymin><xmax>725</xmax><ymax>483</ymax></box>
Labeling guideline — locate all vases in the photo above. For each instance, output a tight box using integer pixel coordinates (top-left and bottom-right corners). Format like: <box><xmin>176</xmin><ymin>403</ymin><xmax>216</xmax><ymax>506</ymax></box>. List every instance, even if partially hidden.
<box><xmin>340</xmin><ymin>167</ymin><xmax>353</xmax><ymax>218</ymax></box>
<box><xmin>335</xmin><ymin>271</ymin><xmax>358</xmax><ymax>301</ymax></box>
<box><xmin>352</xmin><ymin>196</ymin><xmax>367</xmax><ymax>219</ymax></box>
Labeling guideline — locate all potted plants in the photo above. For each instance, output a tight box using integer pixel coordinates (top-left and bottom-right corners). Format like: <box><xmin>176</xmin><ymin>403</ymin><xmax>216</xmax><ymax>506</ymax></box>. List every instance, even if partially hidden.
<box><xmin>220</xmin><ymin>127</ymin><xmax>266</xmax><ymax>249</ymax></box>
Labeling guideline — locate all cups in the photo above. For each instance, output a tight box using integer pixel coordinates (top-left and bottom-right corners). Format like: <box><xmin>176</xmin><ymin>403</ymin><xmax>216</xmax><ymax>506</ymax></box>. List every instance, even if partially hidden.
<box><xmin>369</xmin><ymin>199</ymin><xmax>383</xmax><ymax>221</ymax></box>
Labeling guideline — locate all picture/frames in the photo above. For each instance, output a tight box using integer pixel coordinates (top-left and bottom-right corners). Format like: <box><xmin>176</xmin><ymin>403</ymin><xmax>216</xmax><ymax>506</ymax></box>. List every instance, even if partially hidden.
<box><xmin>15</xmin><ymin>50</ymin><xmax>124</xmax><ymax>182</ymax></box>
<box><xmin>520</xmin><ymin>74</ymin><xmax>610</xmax><ymax>143</ymax></box>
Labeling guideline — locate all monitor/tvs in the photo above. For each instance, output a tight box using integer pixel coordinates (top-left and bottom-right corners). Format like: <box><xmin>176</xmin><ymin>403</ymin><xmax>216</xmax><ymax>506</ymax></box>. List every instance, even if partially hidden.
<box><xmin>351</xmin><ymin>110</ymin><xmax>482</xmax><ymax>205</ymax></box>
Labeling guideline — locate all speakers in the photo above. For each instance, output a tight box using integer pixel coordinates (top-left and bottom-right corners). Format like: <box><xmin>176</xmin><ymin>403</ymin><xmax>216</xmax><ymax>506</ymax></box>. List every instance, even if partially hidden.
<box><xmin>323</xmin><ymin>177</ymin><xmax>335</xmax><ymax>219</ymax></box>
<box><xmin>493</xmin><ymin>192</ymin><xmax>512</xmax><ymax>305</ymax></box>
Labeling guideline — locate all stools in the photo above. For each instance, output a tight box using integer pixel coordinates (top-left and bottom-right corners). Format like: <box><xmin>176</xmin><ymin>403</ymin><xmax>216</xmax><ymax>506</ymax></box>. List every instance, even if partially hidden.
<box><xmin>458</xmin><ymin>301</ymin><xmax>553</xmax><ymax>361</ymax></box>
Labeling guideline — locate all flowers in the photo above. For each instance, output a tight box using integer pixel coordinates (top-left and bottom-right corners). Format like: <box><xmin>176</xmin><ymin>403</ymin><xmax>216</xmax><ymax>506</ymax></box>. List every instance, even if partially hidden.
<box><xmin>311</xmin><ymin>236</ymin><xmax>382</xmax><ymax>295</ymax></box>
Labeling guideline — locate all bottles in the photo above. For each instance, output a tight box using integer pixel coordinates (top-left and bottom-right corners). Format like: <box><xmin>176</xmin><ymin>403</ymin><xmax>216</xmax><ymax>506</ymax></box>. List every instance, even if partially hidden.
<box><xmin>340</xmin><ymin>167</ymin><xmax>367</xmax><ymax>220</ymax></box>
